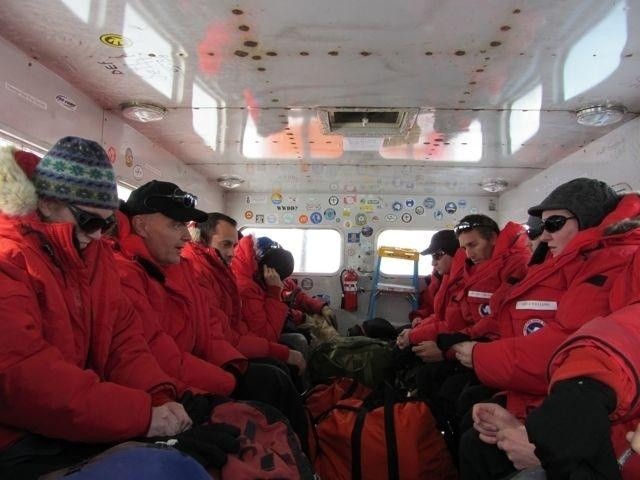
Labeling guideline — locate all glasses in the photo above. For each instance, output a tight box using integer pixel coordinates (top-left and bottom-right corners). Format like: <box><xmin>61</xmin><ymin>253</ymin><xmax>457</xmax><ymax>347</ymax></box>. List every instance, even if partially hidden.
<box><xmin>433</xmin><ymin>249</ymin><xmax>447</xmax><ymax>260</ymax></box>
<box><xmin>454</xmin><ymin>222</ymin><xmax>483</xmax><ymax>234</ymax></box>
<box><xmin>168</xmin><ymin>191</ymin><xmax>198</xmax><ymax>208</ymax></box>
<box><xmin>527</xmin><ymin>231</ymin><xmax>541</xmax><ymax>240</ymax></box>
<box><xmin>540</xmin><ymin>214</ymin><xmax>575</xmax><ymax>232</ymax></box>
<box><xmin>68</xmin><ymin>205</ymin><xmax>118</xmax><ymax>234</ymax></box>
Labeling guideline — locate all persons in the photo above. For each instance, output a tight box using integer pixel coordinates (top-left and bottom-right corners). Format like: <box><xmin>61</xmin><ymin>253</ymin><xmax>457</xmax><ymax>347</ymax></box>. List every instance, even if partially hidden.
<box><xmin>306</xmin><ymin>178</ymin><xmax>640</xmax><ymax>480</ymax></box>
<box><xmin>2</xmin><ymin>135</ymin><xmax>342</xmax><ymax>479</ymax></box>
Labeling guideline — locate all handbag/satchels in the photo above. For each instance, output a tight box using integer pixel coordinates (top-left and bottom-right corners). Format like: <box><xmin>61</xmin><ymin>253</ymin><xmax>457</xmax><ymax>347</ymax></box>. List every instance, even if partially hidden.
<box><xmin>311</xmin><ymin>399</ymin><xmax>449</xmax><ymax>480</ymax></box>
<box><xmin>310</xmin><ymin>334</ymin><xmax>396</xmax><ymax>385</ymax></box>
<box><xmin>301</xmin><ymin>377</ymin><xmax>377</xmax><ymax>416</ymax></box>
<box><xmin>204</xmin><ymin>398</ymin><xmax>309</xmax><ymax>477</ymax></box>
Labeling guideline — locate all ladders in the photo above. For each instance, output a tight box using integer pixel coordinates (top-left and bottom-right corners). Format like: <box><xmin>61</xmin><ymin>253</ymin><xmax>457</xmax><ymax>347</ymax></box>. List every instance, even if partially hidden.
<box><xmin>366</xmin><ymin>245</ymin><xmax>420</xmax><ymax>321</ymax></box>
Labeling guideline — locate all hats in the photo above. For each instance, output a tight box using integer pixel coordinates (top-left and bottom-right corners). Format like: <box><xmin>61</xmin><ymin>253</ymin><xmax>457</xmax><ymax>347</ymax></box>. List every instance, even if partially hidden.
<box><xmin>263</xmin><ymin>248</ymin><xmax>295</xmax><ymax>281</ymax></box>
<box><xmin>127</xmin><ymin>180</ymin><xmax>209</xmax><ymax>222</ymax></box>
<box><xmin>31</xmin><ymin>136</ymin><xmax>119</xmax><ymax>208</ymax></box>
<box><xmin>420</xmin><ymin>230</ymin><xmax>457</xmax><ymax>255</ymax></box>
<box><xmin>253</xmin><ymin>236</ymin><xmax>282</xmax><ymax>260</ymax></box>
<box><xmin>527</xmin><ymin>177</ymin><xmax>620</xmax><ymax>230</ymax></box>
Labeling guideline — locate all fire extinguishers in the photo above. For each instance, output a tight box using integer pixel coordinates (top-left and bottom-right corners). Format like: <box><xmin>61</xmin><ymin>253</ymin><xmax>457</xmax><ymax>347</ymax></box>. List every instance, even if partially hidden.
<box><xmin>339</xmin><ymin>266</ymin><xmax>359</xmax><ymax>312</ymax></box>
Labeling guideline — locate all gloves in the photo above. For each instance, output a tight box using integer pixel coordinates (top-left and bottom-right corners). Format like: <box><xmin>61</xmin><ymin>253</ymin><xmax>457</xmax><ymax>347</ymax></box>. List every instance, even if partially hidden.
<box><xmin>321</xmin><ymin>305</ymin><xmax>339</xmax><ymax>331</ymax></box>
<box><xmin>524</xmin><ymin>377</ymin><xmax>618</xmax><ymax>477</ymax></box>
